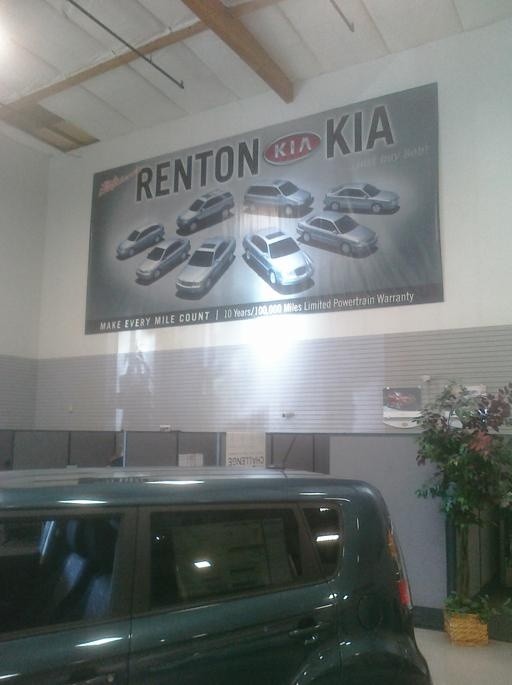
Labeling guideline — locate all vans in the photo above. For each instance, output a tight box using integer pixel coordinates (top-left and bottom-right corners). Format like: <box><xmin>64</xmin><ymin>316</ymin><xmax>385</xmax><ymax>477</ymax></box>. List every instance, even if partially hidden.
<box><xmin>0</xmin><ymin>466</ymin><xmax>434</xmax><ymax>685</ymax></box>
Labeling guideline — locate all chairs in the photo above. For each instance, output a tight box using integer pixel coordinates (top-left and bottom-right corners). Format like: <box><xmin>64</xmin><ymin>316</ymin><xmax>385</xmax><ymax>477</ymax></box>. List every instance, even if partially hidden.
<box><xmin>0</xmin><ymin>521</ymin><xmax>117</xmax><ymax>633</ymax></box>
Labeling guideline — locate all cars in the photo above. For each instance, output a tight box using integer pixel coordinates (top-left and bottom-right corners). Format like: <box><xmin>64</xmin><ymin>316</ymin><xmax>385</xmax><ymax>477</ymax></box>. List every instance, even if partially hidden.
<box><xmin>136</xmin><ymin>235</ymin><xmax>191</xmax><ymax>283</ymax></box>
<box><xmin>117</xmin><ymin>223</ymin><xmax>165</xmax><ymax>258</ymax></box>
<box><xmin>324</xmin><ymin>183</ymin><xmax>401</xmax><ymax>216</ymax></box>
<box><xmin>296</xmin><ymin>211</ymin><xmax>378</xmax><ymax>257</ymax></box>
<box><xmin>387</xmin><ymin>390</ymin><xmax>416</xmax><ymax>410</ymax></box>
<box><xmin>243</xmin><ymin>226</ymin><xmax>314</xmax><ymax>288</ymax></box>
<box><xmin>176</xmin><ymin>235</ymin><xmax>237</xmax><ymax>297</ymax></box>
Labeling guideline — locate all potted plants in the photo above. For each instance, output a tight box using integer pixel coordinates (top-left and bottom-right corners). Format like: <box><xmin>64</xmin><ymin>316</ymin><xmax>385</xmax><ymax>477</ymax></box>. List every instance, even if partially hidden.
<box><xmin>413</xmin><ymin>381</ymin><xmax>512</xmax><ymax>646</ymax></box>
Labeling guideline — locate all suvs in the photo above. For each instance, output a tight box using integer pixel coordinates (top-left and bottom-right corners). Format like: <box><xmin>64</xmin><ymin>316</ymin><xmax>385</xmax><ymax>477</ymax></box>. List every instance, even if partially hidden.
<box><xmin>244</xmin><ymin>178</ymin><xmax>314</xmax><ymax>216</ymax></box>
<box><xmin>177</xmin><ymin>189</ymin><xmax>235</xmax><ymax>234</ymax></box>
